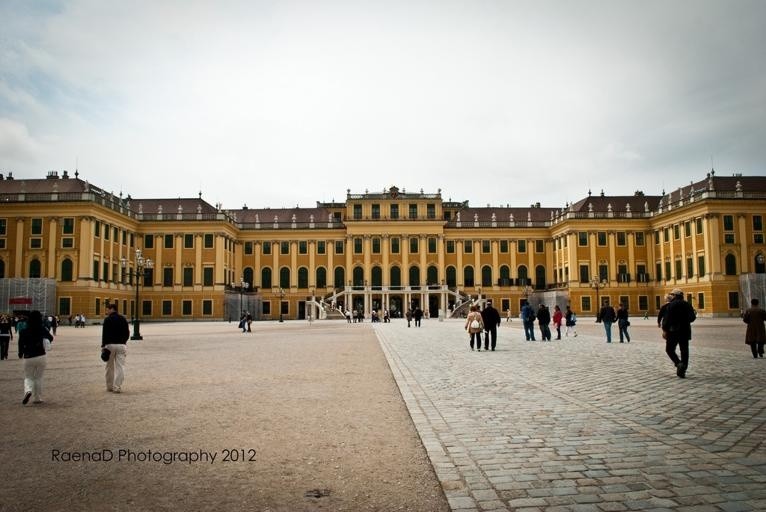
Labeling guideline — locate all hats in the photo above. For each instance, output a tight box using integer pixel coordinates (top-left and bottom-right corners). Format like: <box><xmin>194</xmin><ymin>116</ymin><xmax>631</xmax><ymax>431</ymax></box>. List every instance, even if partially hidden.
<box><xmin>669</xmin><ymin>288</ymin><xmax>684</xmax><ymax>296</ymax></box>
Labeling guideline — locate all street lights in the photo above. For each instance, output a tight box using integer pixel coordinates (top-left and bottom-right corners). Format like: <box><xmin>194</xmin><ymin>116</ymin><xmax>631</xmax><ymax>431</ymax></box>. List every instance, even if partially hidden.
<box><xmin>588</xmin><ymin>276</ymin><xmax>608</xmax><ymax>323</ymax></box>
<box><xmin>520</xmin><ymin>285</ymin><xmax>534</xmax><ymax>305</ymax></box>
<box><xmin>275</xmin><ymin>288</ymin><xmax>285</xmax><ymax>321</ymax></box>
<box><xmin>232</xmin><ymin>275</ymin><xmax>250</xmax><ymax>320</ymax></box>
<box><xmin>119</xmin><ymin>249</ymin><xmax>154</xmax><ymax>343</ymax></box>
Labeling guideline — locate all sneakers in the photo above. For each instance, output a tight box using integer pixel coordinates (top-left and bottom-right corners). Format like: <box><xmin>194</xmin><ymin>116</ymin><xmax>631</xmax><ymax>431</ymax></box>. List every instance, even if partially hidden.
<box><xmin>107</xmin><ymin>386</ymin><xmax>121</xmax><ymax>393</ymax></box>
<box><xmin>759</xmin><ymin>352</ymin><xmax>764</xmax><ymax>357</ymax></box>
<box><xmin>34</xmin><ymin>399</ymin><xmax>44</xmax><ymax>404</ymax></box>
<box><xmin>565</xmin><ymin>334</ymin><xmax>568</xmax><ymax>336</ymax></box>
<box><xmin>677</xmin><ymin>360</ymin><xmax>688</xmax><ymax>378</ymax></box>
<box><xmin>471</xmin><ymin>347</ymin><xmax>496</xmax><ymax>352</ymax></box>
<box><xmin>753</xmin><ymin>353</ymin><xmax>758</xmax><ymax>358</ymax></box>
<box><xmin>22</xmin><ymin>391</ymin><xmax>31</xmax><ymax>406</ymax></box>
<box><xmin>574</xmin><ymin>334</ymin><xmax>578</xmax><ymax>337</ymax></box>
<box><xmin>628</xmin><ymin>337</ymin><xmax>630</xmax><ymax>342</ymax></box>
<box><xmin>526</xmin><ymin>337</ymin><xmax>551</xmax><ymax>341</ymax></box>
<box><xmin>555</xmin><ymin>338</ymin><xmax>561</xmax><ymax>340</ymax></box>
<box><xmin>620</xmin><ymin>341</ymin><xmax>624</xmax><ymax>343</ymax></box>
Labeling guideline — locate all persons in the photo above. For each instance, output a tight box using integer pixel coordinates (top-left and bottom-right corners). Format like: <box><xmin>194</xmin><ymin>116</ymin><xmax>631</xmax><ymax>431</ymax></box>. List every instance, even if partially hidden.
<box><xmin>340</xmin><ymin>299</ymin><xmax>630</xmax><ymax>351</ymax></box>
<box><xmin>247</xmin><ymin>311</ymin><xmax>252</xmax><ymax>332</ymax></box>
<box><xmin>662</xmin><ymin>288</ymin><xmax>696</xmax><ymax>378</ymax></box>
<box><xmin>240</xmin><ymin>309</ymin><xmax>247</xmax><ymax>332</ymax></box>
<box><xmin>101</xmin><ymin>304</ymin><xmax>130</xmax><ymax>393</ymax></box>
<box><xmin>742</xmin><ymin>299</ymin><xmax>766</xmax><ymax>358</ymax></box>
<box><xmin>0</xmin><ymin>310</ymin><xmax>85</xmax><ymax>404</ymax></box>
<box><xmin>657</xmin><ymin>294</ymin><xmax>672</xmax><ymax>327</ymax></box>
<box><xmin>644</xmin><ymin>311</ymin><xmax>648</xmax><ymax>320</ymax></box>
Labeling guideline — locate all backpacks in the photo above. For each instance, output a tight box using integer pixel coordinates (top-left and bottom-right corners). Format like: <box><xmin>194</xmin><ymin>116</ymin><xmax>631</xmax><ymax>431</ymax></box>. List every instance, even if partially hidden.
<box><xmin>470</xmin><ymin>312</ymin><xmax>480</xmax><ymax>330</ymax></box>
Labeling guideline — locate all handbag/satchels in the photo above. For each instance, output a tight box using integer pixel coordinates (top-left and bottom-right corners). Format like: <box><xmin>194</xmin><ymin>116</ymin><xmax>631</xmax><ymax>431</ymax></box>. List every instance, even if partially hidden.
<box><xmin>570</xmin><ymin>313</ymin><xmax>577</xmax><ymax>322</ymax></box>
<box><xmin>100</xmin><ymin>348</ymin><xmax>111</xmax><ymax>362</ymax></box>
